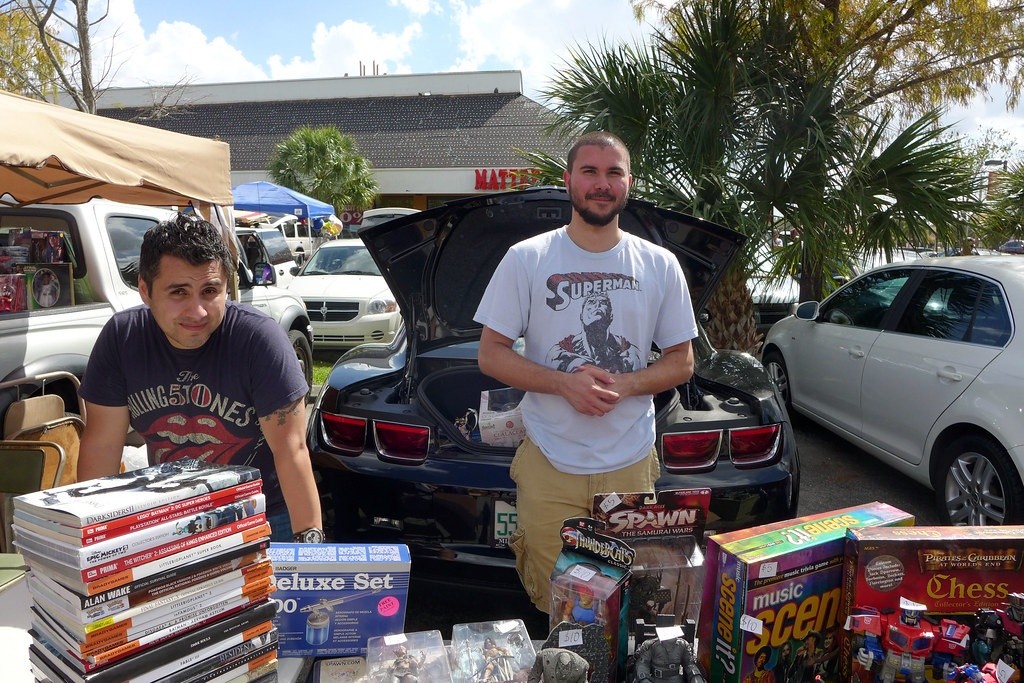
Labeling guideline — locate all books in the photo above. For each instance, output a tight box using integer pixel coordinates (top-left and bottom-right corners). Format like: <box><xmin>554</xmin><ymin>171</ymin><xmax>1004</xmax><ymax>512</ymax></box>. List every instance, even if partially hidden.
<box><xmin>10</xmin><ymin>457</ymin><xmax>279</xmax><ymax>683</ymax></box>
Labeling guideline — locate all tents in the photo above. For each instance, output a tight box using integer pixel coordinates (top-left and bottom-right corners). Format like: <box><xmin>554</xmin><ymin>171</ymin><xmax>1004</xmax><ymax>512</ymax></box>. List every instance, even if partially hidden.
<box><xmin>0</xmin><ymin>90</ymin><xmax>338</xmax><ymax>376</ymax></box>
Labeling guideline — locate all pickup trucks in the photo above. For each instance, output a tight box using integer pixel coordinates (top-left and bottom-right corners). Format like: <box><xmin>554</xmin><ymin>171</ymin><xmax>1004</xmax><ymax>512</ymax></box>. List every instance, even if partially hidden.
<box><xmin>0</xmin><ymin>193</ymin><xmax>315</xmax><ymax>439</ymax></box>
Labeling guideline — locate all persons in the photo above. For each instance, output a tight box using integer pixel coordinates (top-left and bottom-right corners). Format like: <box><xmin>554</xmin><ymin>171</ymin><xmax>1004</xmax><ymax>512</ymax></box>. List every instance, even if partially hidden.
<box><xmin>473</xmin><ymin>130</ymin><xmax>699</xmax><ymax>615</ymax></box>
<box><xmin>73</xmin><ymin>212</ymin><xmax>326</xmax><ymax>546</ymax></box>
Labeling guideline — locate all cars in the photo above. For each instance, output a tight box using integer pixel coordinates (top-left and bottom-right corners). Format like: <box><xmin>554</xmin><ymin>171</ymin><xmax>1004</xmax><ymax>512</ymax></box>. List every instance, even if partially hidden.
<box><xmin>292</xmin><ymin>185</ymin><xmax>802</xmax><ymax>617</ymax></box>
<box><xmin>756</xmin><ymin>253</ymin><xmax>1024</xmax><ymax>527</ymax></box>
<box><xmin>744</xmin><ymin>225</ymin><xmax>999</xmax><ymax>352</ymax></box>
<box><xmin>237</xmin><ymin>205</ymin><xmax>422</xmax><ymax>349</ymax></box>
<box><xmin>999</xmin><ymin>240</ymin><xmax>1024</xmax><ymax>253</ymax></box>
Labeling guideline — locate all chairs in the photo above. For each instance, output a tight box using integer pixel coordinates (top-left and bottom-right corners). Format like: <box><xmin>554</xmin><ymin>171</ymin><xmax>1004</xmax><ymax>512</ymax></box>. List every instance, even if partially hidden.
<box><xmin>0</xmin><ymin>439</ymin><xmax>66</xmax><ymax>595</ymax></box>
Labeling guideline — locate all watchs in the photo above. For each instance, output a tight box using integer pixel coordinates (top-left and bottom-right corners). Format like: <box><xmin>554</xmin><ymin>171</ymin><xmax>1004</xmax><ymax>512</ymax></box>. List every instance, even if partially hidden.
<box><xmin>291</xmin><ymin>527</ymin><xmax>326</xmax><ymax>543</ymax></box>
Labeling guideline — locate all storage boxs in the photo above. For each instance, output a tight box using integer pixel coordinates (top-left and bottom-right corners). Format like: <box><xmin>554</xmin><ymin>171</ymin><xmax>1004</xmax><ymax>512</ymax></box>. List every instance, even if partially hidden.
<box><xmin>838</xmin><ymin>523</ymin><xmax>1024</xmax><ymax>683</ymax></box>
<box><xmin>263</xmin><ymin>543</ymin><xmax>412</xmax><ymax>659</ymax></box>
<box><xmin>548</xmin><ymin>487</ymin><xmax>712</xmax><ymax>683</ymax></box>
<box><xmin>366</xmin><ymin>630</ymin><xmax>454</xmax><ymax>683</ymax></box>
<box><xmin>450</xmin><ymin>619</ymin><xmax>544</xmax><ymax>683</ymax></box>
<box><xmin>479</xmin><ymin>387</ymin><xmax>526</xmax><ymax>448</ymax></box>
<box><xmin>696</xmin><ymin>500</ymin><xmax>915</xmax><ymax>683</ymax></box>
<box><xmin>0</xmin><ymin>227</ymin><xmax>75</xmax><ymax>313</ymax></box>
